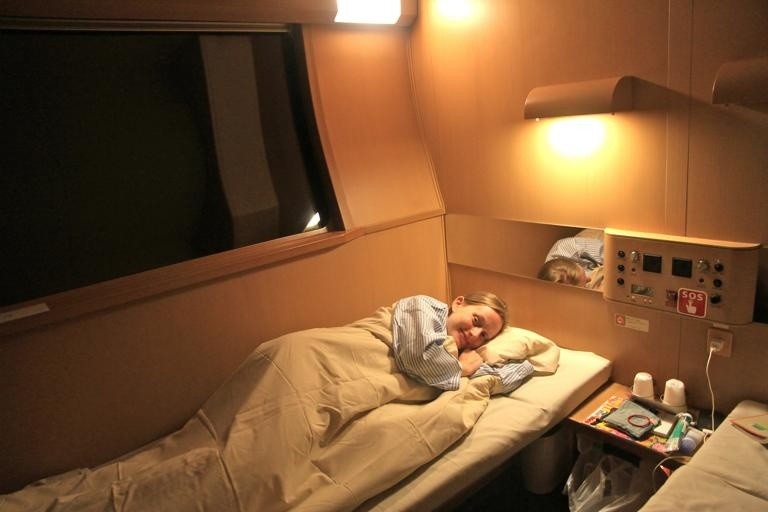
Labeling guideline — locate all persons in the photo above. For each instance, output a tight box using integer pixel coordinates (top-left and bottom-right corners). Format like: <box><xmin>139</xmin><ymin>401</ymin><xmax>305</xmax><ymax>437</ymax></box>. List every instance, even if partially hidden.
<box><xmin>536</xmin><ymin>229</ymin><xmax>605</xmax><ymax>290</ymax></box>
<box><xmin>0</xmin><ymin>291</ymin><xmax>535</xmax><ymax>512</ymax></box>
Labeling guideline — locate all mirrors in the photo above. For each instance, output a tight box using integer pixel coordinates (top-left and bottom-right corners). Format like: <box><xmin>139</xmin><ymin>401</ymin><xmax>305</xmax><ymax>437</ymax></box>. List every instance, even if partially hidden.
<box><xmin>445</xmin><ymin>212</ymin><xmax>603</xmax><ymax>293</ymax></box>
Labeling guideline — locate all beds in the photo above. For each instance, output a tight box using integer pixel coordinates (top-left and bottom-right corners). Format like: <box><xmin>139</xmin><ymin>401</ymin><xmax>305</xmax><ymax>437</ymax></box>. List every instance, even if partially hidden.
<box><xmin>46</xmin><ymin>328</ymin><xmax>613</xmax><ymax>511</ymax></box>
<box><xmin>636</xmin><ymin>397</ymin><xmax>768</xmax><ymax>511</ymax></box>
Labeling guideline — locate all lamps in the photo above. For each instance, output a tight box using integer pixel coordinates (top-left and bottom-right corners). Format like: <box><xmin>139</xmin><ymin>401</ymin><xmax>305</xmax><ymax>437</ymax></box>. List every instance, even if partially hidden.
<box><xmin>524</xmin><ymin>75</ymin><xmax>633</xmax><ymax>120</ymax></box>
<box><xmin>712</xmin><ymin>59</ymin><xmax>767</xmax><ymax>105</ymax></box>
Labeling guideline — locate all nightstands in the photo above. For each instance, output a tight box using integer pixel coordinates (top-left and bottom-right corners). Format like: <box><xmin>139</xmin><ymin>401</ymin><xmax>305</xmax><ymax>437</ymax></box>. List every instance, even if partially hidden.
<box><xmin>567</xmin><ymin>380</ymin><xmax>725</xmax><ymax>466</ymax></box>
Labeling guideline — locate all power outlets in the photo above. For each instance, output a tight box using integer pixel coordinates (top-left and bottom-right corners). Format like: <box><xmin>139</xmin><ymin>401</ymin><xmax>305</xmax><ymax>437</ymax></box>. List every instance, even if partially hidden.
<box><xmin>706</xmin><ymin>329</ymin><xmax>734</xmax><ymax>358</ymax></box>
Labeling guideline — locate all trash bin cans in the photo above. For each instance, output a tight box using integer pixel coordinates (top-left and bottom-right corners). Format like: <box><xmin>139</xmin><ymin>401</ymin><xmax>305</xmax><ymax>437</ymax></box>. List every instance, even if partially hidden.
<box><xmin>522</xmin><ymin>425</ymin><xmax>572</xmax><ymax>495</ymax></box>
<box><xmin>564</xmin><ymin>448</ymin><xmax>655</xmax><ymax>512</ymax></box>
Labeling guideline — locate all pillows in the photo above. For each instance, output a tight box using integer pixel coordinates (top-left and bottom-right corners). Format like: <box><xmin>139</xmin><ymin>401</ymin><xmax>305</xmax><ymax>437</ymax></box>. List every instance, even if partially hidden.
<box><xmin>477</xmin><ymin>328</ymin><xmax>559</xmax><ymax>376</ymax></box>
